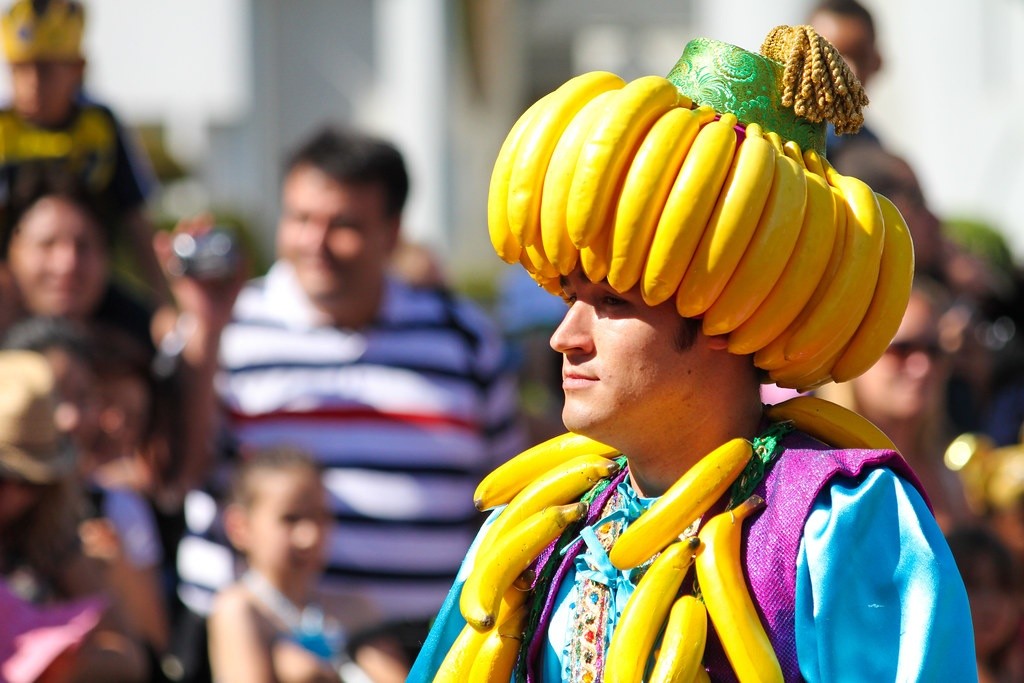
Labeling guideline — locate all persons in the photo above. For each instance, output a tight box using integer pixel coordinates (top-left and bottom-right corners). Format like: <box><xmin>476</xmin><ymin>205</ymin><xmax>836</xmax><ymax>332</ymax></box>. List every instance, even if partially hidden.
<box><xmin>0</xmin><ymin>0</ymin><xmax>570</xmax><ymax>683</ymax></box>
<box><xmin>408</xmin><ymin>27</ymin><xmax>980</xmax><ymax>683</ymax></box>
<box><xmin>758</xmin><ymin>0</ymin><xmax>1023</xmax><ymax>683</ymax></box>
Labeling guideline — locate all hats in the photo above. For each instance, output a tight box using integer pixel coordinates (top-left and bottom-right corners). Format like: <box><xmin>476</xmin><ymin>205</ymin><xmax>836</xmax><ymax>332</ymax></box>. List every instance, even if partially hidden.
<box><xmin>1</xmin><ymin>0</ymin><xmax>86</xmax><ymax>65</ymax></box>
<box><xmin>487</xmin><ymin>25</ymin><xmax>915</xmax><ymax>398</ymax></box>
<box><xmin>0</xmin><ymin>348</ymin><xmax>76</xmax><ymax>485</ymax></box>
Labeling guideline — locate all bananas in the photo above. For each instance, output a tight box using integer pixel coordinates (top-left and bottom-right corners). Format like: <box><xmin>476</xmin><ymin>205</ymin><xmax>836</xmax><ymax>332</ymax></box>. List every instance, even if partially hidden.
<box><xmin>485</xmin><ymin>70</ymin><xmax>915</xmax><ymax>396</ymax></box>
<box><xmin>427</xmin><ymin>394</ymin><xmax>905</xmax><ymax>683</ymax></box>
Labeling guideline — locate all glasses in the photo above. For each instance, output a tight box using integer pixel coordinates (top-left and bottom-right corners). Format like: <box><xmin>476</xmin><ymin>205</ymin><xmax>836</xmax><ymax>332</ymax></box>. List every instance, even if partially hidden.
<box><xmin>876</xmin><ymin>335</ymin><xmax>946</xmax><ymax>371</ymax></box>
